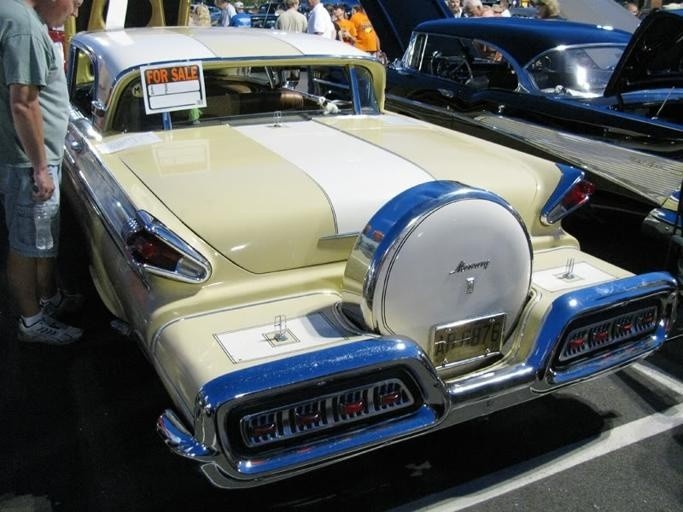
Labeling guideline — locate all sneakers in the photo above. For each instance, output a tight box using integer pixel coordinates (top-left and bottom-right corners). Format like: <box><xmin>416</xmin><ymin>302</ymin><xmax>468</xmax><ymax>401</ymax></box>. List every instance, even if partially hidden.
<box><xmin>39</xmin><ymin>287</ymin><xmax>84</xmax><ymax>316</ymax></box>
<box><xmin>17</xmin><ymin>306</ymin><xmax>85</xmax><ymax>345</ymax></box>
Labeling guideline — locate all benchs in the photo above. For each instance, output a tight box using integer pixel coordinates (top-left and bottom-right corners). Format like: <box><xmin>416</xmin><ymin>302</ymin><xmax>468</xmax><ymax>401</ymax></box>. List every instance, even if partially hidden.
<box><xmin>170</xmin><ymin>90</ymin><xmax>303</xmax><ymax>121</ymax></box>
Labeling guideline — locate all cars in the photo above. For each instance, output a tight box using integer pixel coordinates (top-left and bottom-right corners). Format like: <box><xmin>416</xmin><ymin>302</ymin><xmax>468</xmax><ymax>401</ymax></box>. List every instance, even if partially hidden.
<box><xmin>313</xmin><ymin>1</ymin><xmax>681</xmax><ymax>247</ymax></box>
<box><xmin>55</xmin><ymin>0</ymin><xmax>682</xmax><ymax>494</ymax></box>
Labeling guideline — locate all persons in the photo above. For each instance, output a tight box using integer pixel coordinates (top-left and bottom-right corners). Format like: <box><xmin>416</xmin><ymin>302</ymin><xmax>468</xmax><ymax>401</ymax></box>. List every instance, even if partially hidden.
<box><xmin>189</xmin><ymin>3</ymin><xmax>212</xmax><ymax>27</ymax></box>
<box><xmin>625</xmin><ymin>3</ymin><xmax>640</xmax><ymax>19</ymax></box>
<box><xmin>0</xmin><ymin>1</ymin><xmax>87</xmax><ymax>346</ymax></box>
<box><xmin>213</xmin><ymin>0</ymin><xmax>510</xmax><ymax>62</ymax></box>
<box><xmin>535</xmin><ymin>0</ymin><xmax>564</xmax><ymax>19</ymax></box>
